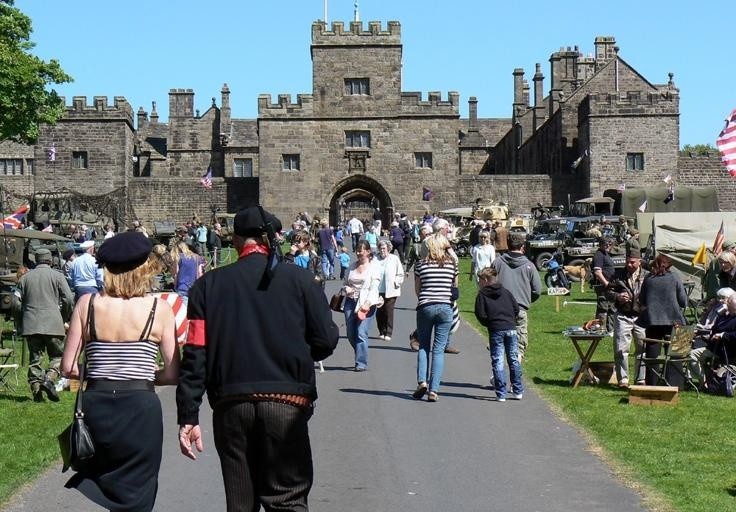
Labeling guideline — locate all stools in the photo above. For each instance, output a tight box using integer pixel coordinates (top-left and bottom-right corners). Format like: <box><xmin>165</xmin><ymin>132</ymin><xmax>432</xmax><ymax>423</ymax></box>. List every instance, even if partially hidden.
<box><xmin>0</xmin><ymin>361</ymin><xmax>22</xmax><ymax>396</ymax></box>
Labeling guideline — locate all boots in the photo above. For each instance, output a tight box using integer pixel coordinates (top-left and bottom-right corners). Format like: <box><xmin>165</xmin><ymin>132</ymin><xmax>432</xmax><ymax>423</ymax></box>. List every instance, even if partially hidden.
<box><xmin>40</xmin><ymin>368</ymin><xmax>62</xmax><ymax>403</ymax></box>
<box><xmin>31</xmin><ymin>381</ymin><xmax>46</xmax><ymax>402</ymax></box>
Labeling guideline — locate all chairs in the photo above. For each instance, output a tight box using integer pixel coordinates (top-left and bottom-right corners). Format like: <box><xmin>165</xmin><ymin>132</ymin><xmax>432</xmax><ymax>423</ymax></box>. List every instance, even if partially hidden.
<box><xmin>0</xmin><ymin>329</ymin><xmax>19</xmax><ymax>386</ymax></box>
<box><xmin>634</xmin><ymin>323</ymin><xmax>699</xmax><ymax>399</ymax></box>
<box><xmin>679</xmin><ymin>279</ymin><xmax>736</xmax><ymax>398</ymax></box>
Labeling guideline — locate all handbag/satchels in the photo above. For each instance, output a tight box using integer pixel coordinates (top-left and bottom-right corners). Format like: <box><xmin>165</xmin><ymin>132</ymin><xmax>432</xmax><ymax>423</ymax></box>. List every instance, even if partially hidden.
<box><xmin>55</xmin><ymin>412</ymin><xmax>96</xmax><ymax>476</ymax></box>
<box><xmin>328</xmin><ymin>287</ymin><xmax>345</xmax><ymax>314</ymax></box>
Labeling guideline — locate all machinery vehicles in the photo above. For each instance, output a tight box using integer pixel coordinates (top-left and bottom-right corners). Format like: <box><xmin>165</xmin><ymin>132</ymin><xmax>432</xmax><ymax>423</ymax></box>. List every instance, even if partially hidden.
<box><xmin>210</xmin><ymin>212</ymin><xmax>237</xmax><ymax>249</ymax></box>
<box><xmin>0</xmin><ymin>194</ymin><xmax>113</xmax><ymax>314</ymax></box>
<box><xmin>435</xmin><ymin>195</ymin><xmax>651</xmax><ymax>283</ymax></box>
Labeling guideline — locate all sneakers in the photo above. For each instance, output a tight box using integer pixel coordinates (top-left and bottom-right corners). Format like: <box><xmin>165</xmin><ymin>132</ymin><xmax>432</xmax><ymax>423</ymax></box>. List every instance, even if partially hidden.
<box><xmin>443</xmin><ymin>345</ymin><xmax>461</xmax><ymax>354</ymax></box>
<box><xmin>512</xmin><ymin>391</ymin><xmax>524</xmax><ymax>400</ymax></box>
<box><xmin>427</xmin><ymin>391</ymin><xmax>440</xmax><ymax>402</ymax></box>
<box><xmin>384</xmin><ymin>336</ymin><xmax>392</xmax><ymax>342</ymax></box>
<box><xmin>378</xmin><ymin>334</ymin><xmax>386</xmax><ymax>340</ymax></box>
<box><xmin>408</xmin><ymin>333</ymin><xmax>420</xmax><ymax>352</ymax></box>
<box><xmin>412</xmin><ymin>381</ymin><xmax>428</xmax><ymax>400</ymax></box>
<box><xmin>618</xmin><ymin>382</ymin><xmax>648</xmax><ymax>389</ymax></box>
<box><xmin>488</xmin><ymin>375</ymin><xmax>496</xmax><ymax>386</ymax></box>
<box><xmin>495</xmin><ymin>395</ymin><xmax>507</xmax><ymax>403</ymax></box>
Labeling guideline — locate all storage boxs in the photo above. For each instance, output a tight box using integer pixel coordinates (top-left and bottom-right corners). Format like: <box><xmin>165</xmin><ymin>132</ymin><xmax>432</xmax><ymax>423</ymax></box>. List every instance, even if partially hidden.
<box><xmin>627</xmin><ymin>383</ymin><xmax>679</xmax><ymax>408</ymax></box>
<box><xmin>589</xmin><ymin>359</ymin><xmax>619</xmax><ymax>386</ymax></box>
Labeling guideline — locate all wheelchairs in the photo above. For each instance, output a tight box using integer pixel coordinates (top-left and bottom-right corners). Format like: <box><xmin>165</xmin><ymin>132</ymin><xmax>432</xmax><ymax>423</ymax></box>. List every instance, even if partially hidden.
<box><xmin>682</xmin><ymin>338</ymin><xmax>736</xmax><ymax>393</ymax></box>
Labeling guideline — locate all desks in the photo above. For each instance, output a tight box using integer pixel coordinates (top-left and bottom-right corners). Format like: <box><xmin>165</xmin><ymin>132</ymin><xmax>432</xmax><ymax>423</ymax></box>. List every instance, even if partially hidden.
<box><xmin>561</xmin><ymin>324</ymin><xmax>608</xmax><ymax>391</ymax></box>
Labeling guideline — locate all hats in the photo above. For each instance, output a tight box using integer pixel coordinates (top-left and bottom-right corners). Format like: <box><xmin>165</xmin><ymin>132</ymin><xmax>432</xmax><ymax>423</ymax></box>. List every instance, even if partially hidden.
<box><xmin>95</xmin><ymin>230</ymin><xmax>154</xmax><ymax>276</ymax></box>
<box><xmin>35</xmin><ymin>248</ymin><xmax>54</xmax><ymax>266</ymax></box>
<box><xmin>720</xmin><ymin>240</ymin><xmax>736</xmax><ymax>252</ymax></box>
<box><xmin>388</xmin><ymin>211</ymin><xmax>409</xmax><ymax>230</ymax></box>
<box><xmin>234</xmin><ymin>206</ymin><xmax>282</xmax><ymax>236</ymax></box>
<box><xmin>625</xmin><ymin>246</ymin><xmax>641</xmax><ymax>259</ymax></box>
<box><xmin>79</xmin><ymin>239</ymin><xmax>96</xmax><ymax>251</ymax></box>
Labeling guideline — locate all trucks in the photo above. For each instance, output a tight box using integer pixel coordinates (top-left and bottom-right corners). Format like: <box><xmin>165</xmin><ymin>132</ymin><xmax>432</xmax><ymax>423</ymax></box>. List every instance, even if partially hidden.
<box><xmin>569</xmin><ymin>185</ymin><xmax>719</xmax><ymax>230</ymax></box>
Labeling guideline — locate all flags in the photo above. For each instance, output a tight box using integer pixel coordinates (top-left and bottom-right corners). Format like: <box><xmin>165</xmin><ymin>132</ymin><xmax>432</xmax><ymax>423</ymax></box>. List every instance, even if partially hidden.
<box><xmin>200</xmin><ymin>168</ymin><xmax>213</xmax><ymax>190</ymax></box>
<box><xmin>0</xmin><ymin>205</ymin><xmax>31</xmax><ymax>229</ymax></box>
<box><xmin>716</xmin><ymin>109</ymin><xmax>736</xmax><ymax>178</ymax></box>
<box><xmin>422</xmin><ymin>187</ymin><xmax>437</xmax><ymax>201</ymax></box>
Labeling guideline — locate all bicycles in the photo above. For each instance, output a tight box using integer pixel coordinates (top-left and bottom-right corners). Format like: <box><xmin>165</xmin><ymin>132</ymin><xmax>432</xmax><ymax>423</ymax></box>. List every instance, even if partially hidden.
<box><xmin>692</xmin><ymin>295</ymin><xmax>711</xmax><ymax>325</ymax></box>
